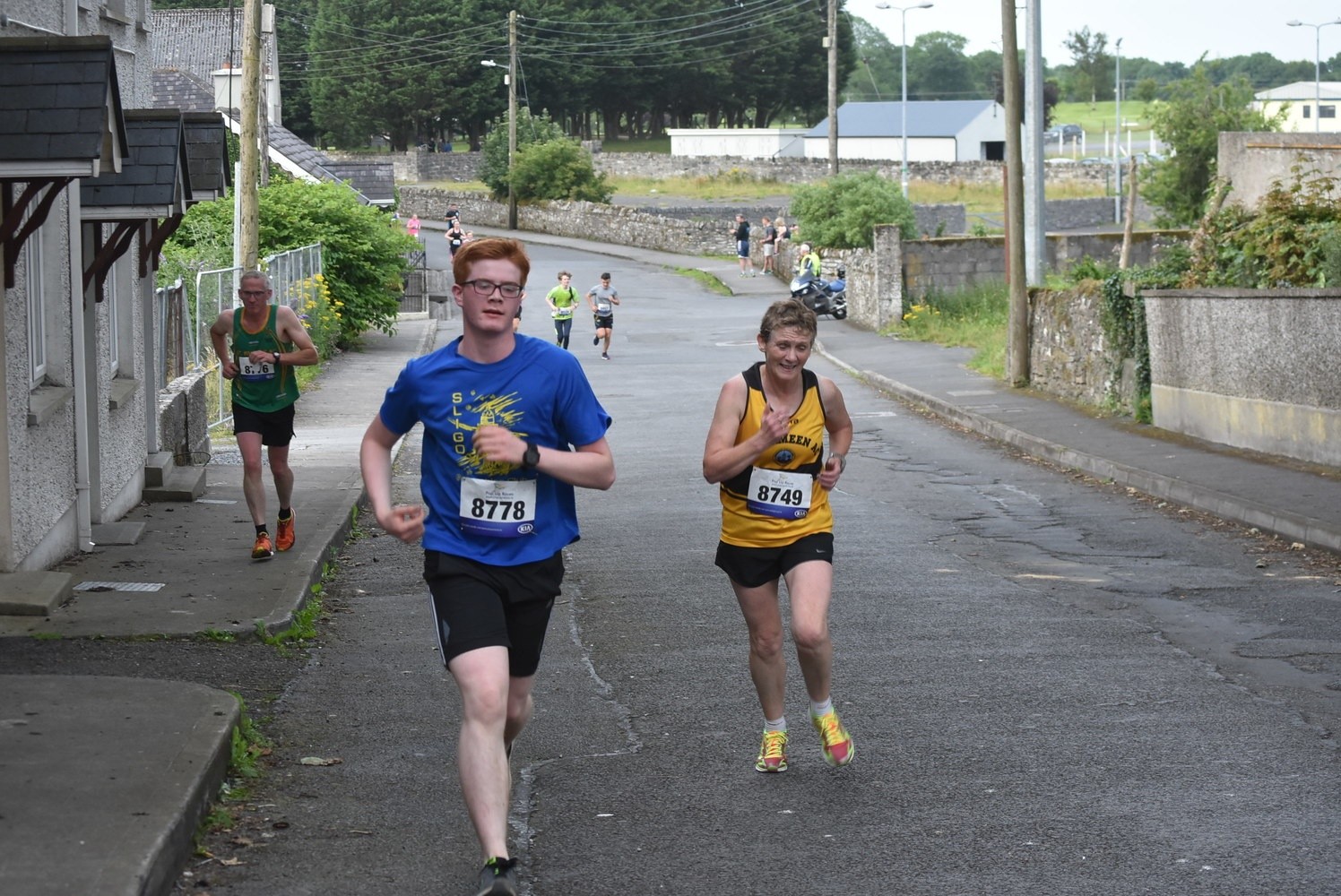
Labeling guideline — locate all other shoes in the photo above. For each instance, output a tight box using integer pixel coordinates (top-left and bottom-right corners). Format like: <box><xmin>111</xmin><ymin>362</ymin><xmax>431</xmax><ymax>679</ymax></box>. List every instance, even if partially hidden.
<box><xmin>760</xmin><ymin>270</ymin><xmax>768</xmax><ymax>275</ymax></box>
<box><xmin>740</xmin><ymin>273</ymin><xmax>747</xmax><ymax>278</ymax></box>
<box><xmin>750</xmin><ymin>272</ymin><xmax>756</xmax><ymax>276</ymax></box>
<box><xmin>769</xmin><ymin>271</ymin><xmax>774</xmax><ymax>274</ymax></box>
<box><xmin>774</xmin><ymin>252</ymin><xmax>780</xmax><ymax>256</ymax></box>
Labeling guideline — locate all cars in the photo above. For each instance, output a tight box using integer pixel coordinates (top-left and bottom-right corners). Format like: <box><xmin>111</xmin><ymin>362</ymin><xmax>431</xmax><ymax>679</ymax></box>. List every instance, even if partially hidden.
<box><xmin>365</xmin><ymin>199</ymin><xmax>397</xmax><ymax>216</ymax></box>
<box><xmin>1043</xmin><ymin>140</ymin><xmax>1175</xmax><ymax>169</ymax></box>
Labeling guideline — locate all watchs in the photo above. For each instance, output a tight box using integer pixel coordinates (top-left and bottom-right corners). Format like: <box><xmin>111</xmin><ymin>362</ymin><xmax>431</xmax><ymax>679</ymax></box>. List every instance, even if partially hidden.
<box><xmin>272</xmin><ymin>352</ymin><xmax>280</xmax><ymax>365</ymax></box>
<box><xmin>828</xmin><ymin>453</ymin><xmax>846</xmax><ymax>474</ymax></box>
<box><xmin>521</xmin><ymin>440</ymin><xmax>540</xmax><ymax>469</ymax></box>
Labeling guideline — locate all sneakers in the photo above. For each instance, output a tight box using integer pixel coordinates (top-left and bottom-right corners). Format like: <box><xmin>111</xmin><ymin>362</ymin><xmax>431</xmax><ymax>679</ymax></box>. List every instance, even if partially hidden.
<box><xmin>251</xmin><ymin>532</ymin><xmax>274</xmax><ymax>559</ymax></box>
<box><xmin>275</xmin><ymin>506</ymin><xmax>296</xmax><ymax>551</ymax></box>
<box><xmin>476</xmin><ymin>855</ymin><xmax>519</xmax><ymax>895</ymax></box>
<box><xmin>755</xmin><ymin>727</ymin><xmax>788</xmax><ymax>772</ymax></box>
<box><xmin>602</xmin><ymin>353</ymin><xmax>609</xmax><ymax>360</ymax></box>
<box><xmin>593</xmin><ymin>336</ymin><xmax>599</xmax><ymax>345</ymax></box>
<box><xmin>557</xmin><ymin>341</ymin><xmax>562</xmax><ymax>347</ymax></box>
<box><xmin>505</xmin><ymin>743</ymin><xmax>513</xmax><ymax>787</ymax></box>
<box><xmin>806</xmin><ymin>701</ymin><xmax>855</xmax><ymax>767</ymax></box>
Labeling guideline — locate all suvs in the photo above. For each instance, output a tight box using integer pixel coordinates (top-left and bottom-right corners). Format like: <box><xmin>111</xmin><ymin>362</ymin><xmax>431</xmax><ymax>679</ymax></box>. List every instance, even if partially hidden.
<box><xmin>1043</xmin><ymin>123</ymin><xmax>1083</xmax><ymax>146</ymax></box>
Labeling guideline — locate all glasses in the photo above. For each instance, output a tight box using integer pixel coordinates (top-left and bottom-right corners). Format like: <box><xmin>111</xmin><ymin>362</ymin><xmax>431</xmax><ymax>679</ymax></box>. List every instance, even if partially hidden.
<box><xmin>459</xmin><ymin>278</ymin><xmax>523</xmax><ymax>299</ymax></box>
<box><xmin>240</xmin><ymin>288</ymin><xmax>267</xmax><ymax>300</ymax></box>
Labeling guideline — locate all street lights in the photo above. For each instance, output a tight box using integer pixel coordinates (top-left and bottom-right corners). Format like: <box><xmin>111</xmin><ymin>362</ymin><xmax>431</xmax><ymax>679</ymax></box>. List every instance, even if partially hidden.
<box><xmin>480</xmin><ymin>59</ymin><xmax>520</xmax><ymax>231</ymax></box>
<box><xmin>1287</xmin><ymin>18</ymin><xmax>1341</xmax><ymax>133</ymax></box>
<box><xmin>1114</xmin><ymin>36</ymin><xmax>1124</xmax><ymax>226</ymax></box>
<box><xmin>878</xmin><ymin>4</ymin><xmax>935</xmax><ymax>201</ymax></box>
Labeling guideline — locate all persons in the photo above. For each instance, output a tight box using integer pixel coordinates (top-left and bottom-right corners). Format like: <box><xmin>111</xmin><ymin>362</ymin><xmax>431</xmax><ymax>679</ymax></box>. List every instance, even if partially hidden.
<box><xmin>209</xmin><ymin>270</ymin><xmax>319</xmax><ymax>558</ymax></box>
<box><xmin>388</xmin><ymin>203</ymin><xmax>473</xmax><ymax>256</ymax></box>
<box><xmin>508</xmin><ymin>285</ymin><xmax>526</xmax><ymax>334</ymax></box>
<box><xmin>546</xmin><ymin>271</ymin><xmax>580</xmax><ymax>351</ymax></box>
<box><xmin>586</xmin><ymin>272</ymin><xmax>620</xmax><ymax>360</ymax></box>
<box><xmin>729</xmin><ymin>213</ymin><xmax>823</xmax><ymax>281</ymax></box>
<box><xmin>359</xmin><ymin>238</ymin><xmax>616</xmax><ymax>894</ymax></box>
<box><xmin>702</xmin><ymin>299</ymin><xmax>855</xmax><ymax>775</ymax></box>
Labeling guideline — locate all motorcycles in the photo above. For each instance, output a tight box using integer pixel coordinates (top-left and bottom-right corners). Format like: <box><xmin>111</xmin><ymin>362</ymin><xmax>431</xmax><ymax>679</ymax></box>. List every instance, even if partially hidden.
<box><xmin>789</xmin><ymin>258</ymin><xmax>848</xmax><ymax>321</ymax></box>
